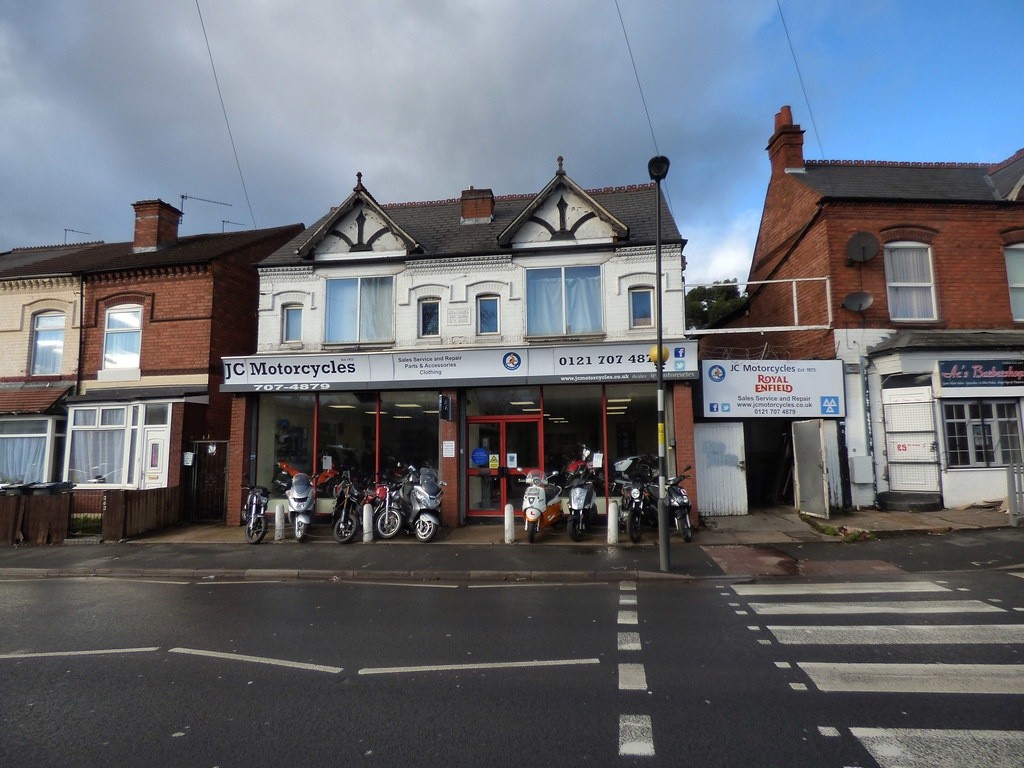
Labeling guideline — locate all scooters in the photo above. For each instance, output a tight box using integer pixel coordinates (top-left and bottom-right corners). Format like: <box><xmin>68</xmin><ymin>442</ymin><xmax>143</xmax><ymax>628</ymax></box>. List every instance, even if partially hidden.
<box><xmin>515</xmin><ymin>466</ymin><xmax>564</xmax><ymax>543</ymax></box>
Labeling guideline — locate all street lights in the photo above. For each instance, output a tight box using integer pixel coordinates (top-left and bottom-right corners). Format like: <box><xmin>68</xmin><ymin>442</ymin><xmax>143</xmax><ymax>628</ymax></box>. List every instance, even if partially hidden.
<box><xmin>647</xmin><ymin>155</ymin><xmax>671</xmax><ymax>569</ymax></box>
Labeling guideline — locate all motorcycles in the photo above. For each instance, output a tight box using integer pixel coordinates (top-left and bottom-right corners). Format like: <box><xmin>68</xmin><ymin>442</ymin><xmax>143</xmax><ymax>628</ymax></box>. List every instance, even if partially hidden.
<box><xmin>618</xmin><ymin>477</ymin><xmax>650</xmax><ymax>542</ymax></box>
<box><xmin>272</xmin><ymin>450</ymin><xmax>345</xmax><ymax>499</ymax></box>
<box><xmin>396</xmin><ymin>461</ymin><xmax>448</xmax><ymax>543</ymax></box>
<box><xmin>596</xmin><ymin>454</ymin><xmax>657</xmax><ymax>495</ymax></box>
<box><xmin>356</xmin><ymin>471</ymin><xmax>402</xmax><ymax>538</ymax></box>
<box><xmin>238</xmin><ymin>473</ymin><xmax>271</xmax><ymax>544</ymax></box>
<box><xmin>331</xmin><ymin>465</ymin><xmax>367</xmax><ymax>544</ymax></box>
<box><xmin>648</xmin><ymin>465</ymin><xmax>692</xmax><ymax>542</ymax></box>
<box><xmin>285</xmin><ymin>473</ymin><xmax>319</xmax><ymax>542</ymax></box>
<box><xmin>563</xmin><ymin>447</ymin><xmax>598</xmax><ymax>541</ymax></box>
